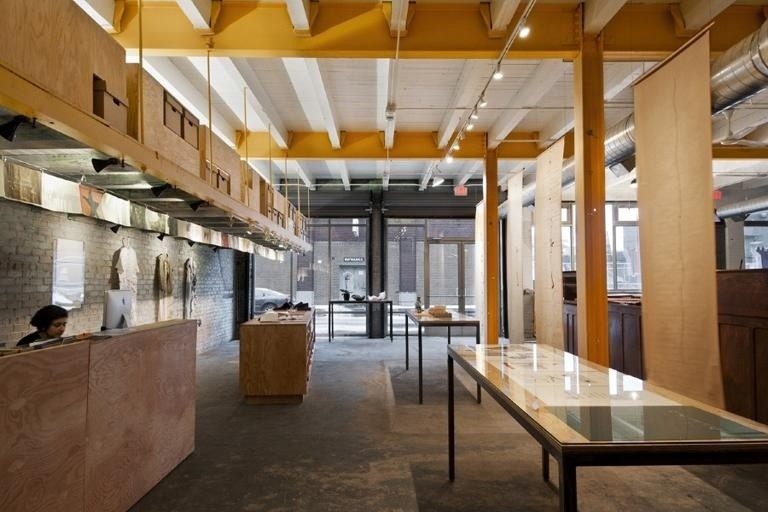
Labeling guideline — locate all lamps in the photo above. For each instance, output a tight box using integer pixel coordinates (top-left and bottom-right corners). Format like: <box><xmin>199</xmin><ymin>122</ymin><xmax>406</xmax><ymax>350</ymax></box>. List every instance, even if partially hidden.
<box><xmin>430</xmin><ymin>18</ymin><xmax>539</xmax><ymax>188</ymax></box>
<box><xmin>109</xmin><ymin>221</ymin><xmax>222</xmax><ymax>253</ymax></box>
<box><xmin>1</xmin><ymin>112</ymin><xmax>208</xmax><ymax>213</ymax></box>
<box><xmin>607</xmin><ymin>156</ymin><xmax>635</xmax><ymax>178</ymax></box>
<box><xmin>245</xmin><ymin>221</ymin><xmax>304</xmax><ymax>258</ymax></box>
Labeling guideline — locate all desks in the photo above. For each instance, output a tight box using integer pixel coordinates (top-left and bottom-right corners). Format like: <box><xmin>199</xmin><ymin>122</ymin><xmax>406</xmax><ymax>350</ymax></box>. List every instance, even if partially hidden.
<box><xmin>328</xmin><ymin>299</ymin><xmax>394</xmax><ymax>343</ymax></box>
<box><xmin>445</xmin><ymin>340</ymin><xmax>768</xmax><ymax>511</ymax></box>
<box><xmin>404</xmin><ymin>307</ymin><xmax>482</xmax><ymax>406</ymax></box>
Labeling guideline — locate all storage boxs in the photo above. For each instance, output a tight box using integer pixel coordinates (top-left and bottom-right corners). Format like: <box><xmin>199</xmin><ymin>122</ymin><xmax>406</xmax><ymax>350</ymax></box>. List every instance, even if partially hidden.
<box><xmin>268</xmin><ymin>194</ymin><xmax>308</xmax><ymax>241</ymax></box>
<box><xmin>218</xmin><ymin>170</ymin><xmax>229</xmax><ymax>194</ymax></box>
<box><xmin>163</xmin><ymin>89</ymin><xmax>184</xmax><ymax>138</ymax></box>
<box><xmin>205</xmin><ymin>160</ymin><xmax>217</xmax><ymax>188</ymax></box>
<box><xmin>94</xmin><ymin>78</ymin><xmax>131</xmax><ymax>134</ymax></box>
<box><xmin>183</xmin><ymin>107</ymin><xmax>199</xmax><ymax>150</ymax></box>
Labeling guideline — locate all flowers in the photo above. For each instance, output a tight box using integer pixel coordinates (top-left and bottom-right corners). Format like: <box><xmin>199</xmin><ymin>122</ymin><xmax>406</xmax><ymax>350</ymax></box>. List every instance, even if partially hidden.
<box><xmin>338</xmin><ymin>272</ymin><xmax>354</xmax><ymax>293</ymax></box>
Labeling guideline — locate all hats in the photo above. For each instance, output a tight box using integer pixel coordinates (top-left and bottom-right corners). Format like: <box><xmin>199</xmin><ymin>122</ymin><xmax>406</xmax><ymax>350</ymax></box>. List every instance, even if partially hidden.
<box><xmin>29</xmin><ymin>305</ymin><xmax>69</xmax><ymax>333</ymax></box>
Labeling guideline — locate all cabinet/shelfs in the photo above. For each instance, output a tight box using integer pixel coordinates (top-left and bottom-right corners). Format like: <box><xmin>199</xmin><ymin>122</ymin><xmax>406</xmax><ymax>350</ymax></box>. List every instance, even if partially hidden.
<box><xmin>239</xmin><ymin>305</ymin><xmax>317</xmax><ymax>405</ymax></box>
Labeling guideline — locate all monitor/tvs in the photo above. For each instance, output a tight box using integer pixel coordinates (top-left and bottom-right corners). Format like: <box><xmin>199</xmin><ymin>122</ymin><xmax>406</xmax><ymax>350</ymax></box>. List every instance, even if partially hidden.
<box><xmin>103</xmin><ymin>290</ymin><xmax>133</xmax><ymax>329</ymax></box>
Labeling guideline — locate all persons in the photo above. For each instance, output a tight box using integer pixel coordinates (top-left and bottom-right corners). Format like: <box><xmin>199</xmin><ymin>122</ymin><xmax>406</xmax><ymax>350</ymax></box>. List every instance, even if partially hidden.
<box><xmin>17</xmin><ymin>305</ymin><xmax>67</xmax><ymax>346</ymax></box>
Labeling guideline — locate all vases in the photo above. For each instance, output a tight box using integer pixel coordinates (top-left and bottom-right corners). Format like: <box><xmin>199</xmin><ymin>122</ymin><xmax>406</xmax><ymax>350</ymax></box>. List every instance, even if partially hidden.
<box><xmin>344</xmin><ymin>292</ymin><xmax>350</xmax><ymax>301</ymax></box>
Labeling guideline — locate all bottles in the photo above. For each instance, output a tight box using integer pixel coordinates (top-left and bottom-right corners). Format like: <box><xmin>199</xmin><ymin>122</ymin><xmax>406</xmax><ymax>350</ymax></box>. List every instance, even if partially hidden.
<box><xmin>416</xmin><ymin>296</ymin><xmax>422</xmax><ymax>313</ymax></box>
